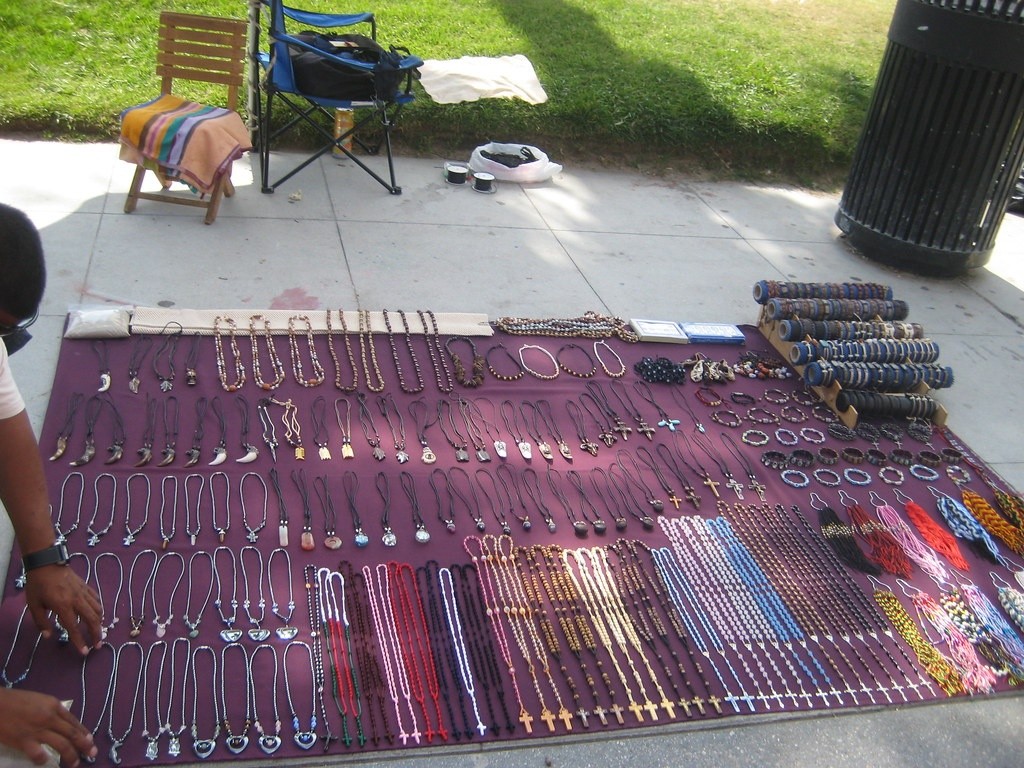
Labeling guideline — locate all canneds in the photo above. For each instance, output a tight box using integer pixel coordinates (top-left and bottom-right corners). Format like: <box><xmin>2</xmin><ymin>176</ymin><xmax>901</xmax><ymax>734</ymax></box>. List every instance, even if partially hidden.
<box><xmin>332</xmin><ymin>106</ymin><xmax>354</xmax><ymax>160</ymax></box>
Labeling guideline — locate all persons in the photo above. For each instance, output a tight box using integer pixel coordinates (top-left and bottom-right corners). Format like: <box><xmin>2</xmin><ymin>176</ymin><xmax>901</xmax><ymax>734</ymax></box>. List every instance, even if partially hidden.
<box><xmin>0</xmin><ymin>203</ymin><xmax>104</xmax><ymax>768</ymax></box>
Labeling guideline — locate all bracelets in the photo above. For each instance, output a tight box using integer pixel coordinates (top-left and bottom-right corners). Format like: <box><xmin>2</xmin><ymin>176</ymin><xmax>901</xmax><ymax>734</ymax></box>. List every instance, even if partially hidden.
<box><xmin>592</xmin><ymin>339</ymin><xmax>626</xmax><ymax>377</ymax></box>
<box><xmin>446</xmin><ymin>336</ymin><xmax>484</xmax><ymax>388</ymax></box>
<box><xmin>556</xmin><ymin>343</ymin><xmax>596</xmax><ymax>379</ymax></box>
<box><xmin>486</xmin><ymin>342</ymin><xmax>525</xmax><ymax>380</ymax></box>
<box><xmin>634</xmin><ymin>280</ymin><xmax>974</xmax><ymax>486</ymax></box>
<box><xmin>519</xmin><ymin>344</ymin><xmax>560</xmax><ymax>380</ymax></box>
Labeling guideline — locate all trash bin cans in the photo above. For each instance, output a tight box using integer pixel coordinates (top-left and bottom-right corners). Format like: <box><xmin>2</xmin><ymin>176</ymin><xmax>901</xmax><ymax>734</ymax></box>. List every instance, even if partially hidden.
<box><xmin>834</xmin><ymin>0</ymin><xmax>1024</xmax><ymax>276</ymax></box>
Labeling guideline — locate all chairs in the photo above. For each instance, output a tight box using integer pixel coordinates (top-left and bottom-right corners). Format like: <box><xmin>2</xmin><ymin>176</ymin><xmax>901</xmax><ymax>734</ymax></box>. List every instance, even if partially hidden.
<box><xmin>121</xmin><ymin>12</ymin><xmax>247</xmax><ymax>225</ymax></box>
<box><xmin>251</xmin><ymin>0</ymin><xmax>424</xmax><ymax>194</ymax></box>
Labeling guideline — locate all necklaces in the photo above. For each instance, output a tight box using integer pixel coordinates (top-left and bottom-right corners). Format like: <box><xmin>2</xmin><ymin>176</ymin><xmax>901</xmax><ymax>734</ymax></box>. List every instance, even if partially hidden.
<box><xmin>154</xmin><ymin>321</ymin><xmax>182</xmax><ymax>392</ymax></box>
<box><xmin>418</xmin><ymin>311</ymin><xmax>459</xmax><ymax>394</ymax></box>
<box><xmin>4</xmin><ymin>602</ymin><xmax>53</xmax><ymax>690</ymax></box>
<box><xmin>249</xmin><ymin>313</ymin><xmax>286</xmax><ymax>390</ymax></box>
<box><xmin>128</xmin><ymin>336</ymin><xmax>153</xmax><ymax>393</ymax></box>
<box><xmin>383</xmin><ymin>309</ymin><xmax>425</xmax><ymax>392</ymax></box>
<box><xmin>185</xmin><ymin>331</ymin><xmax>202</xmax><ymax>385</ymax></box>
<box><xmin>327</xmin><ymin>308</ymin><xmax>358</xmax><ymax>392</ymax></box>
<box><xmin>289</xmin><ymin>314</ymin><xmax>326</xmax><ymax>387</ymax></box>
<box><xmin>358</xmin><ymin>309</ymin><xmax>385</xmax><ymax>391</ymax></box>
<box><xmin>53</xmin><ymin>470</ymin><xmax>85</xmax><ymax>546</ymax></box>
<box><xmin>20</xmin><ymin>383</ymin><xmax>936</xmax><ymax>766</ymax></box>
<box><xmin>489</xmin><ymin>309</ymin><xmax>639</xmax><ymax>343</ymax></box>
<box><xmin>213</xmin><ymin>313</ymin><xmax>245</xmax><ymax>390</ymax></box>
<box><xmin>91</xmin><ymin>337</ymin><xmax>111</xmax><ymax>392</ymax></box>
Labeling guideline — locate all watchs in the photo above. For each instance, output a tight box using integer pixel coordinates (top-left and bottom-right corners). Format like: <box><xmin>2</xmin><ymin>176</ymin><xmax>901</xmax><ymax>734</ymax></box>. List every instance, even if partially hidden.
<box><xmin>22</xmin><ymin>539</ymin><xmax>70</xmax><ymax>571</ymax></box>
